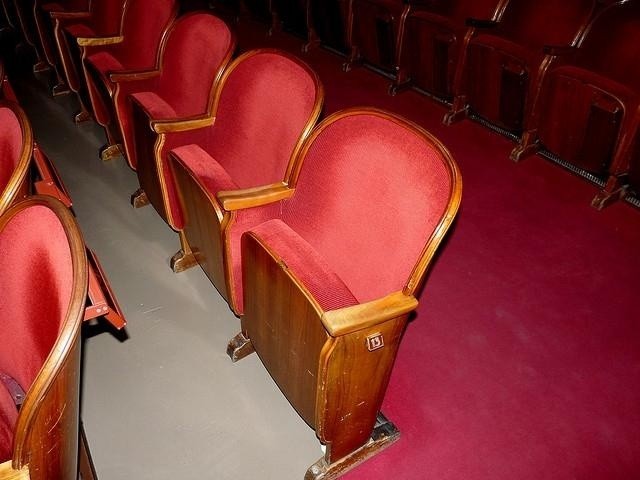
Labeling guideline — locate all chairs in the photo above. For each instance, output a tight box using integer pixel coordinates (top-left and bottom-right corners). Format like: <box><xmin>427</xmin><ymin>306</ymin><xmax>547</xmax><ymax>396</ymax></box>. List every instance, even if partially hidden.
<box><xmin>0</xmin><ymin>66</ymin><xmax>94</xmax><ymax>480</ymax></box>
<box><xmin>248</xmin><ymin>2</ymin><xmax>640</xmax><ymax>218</ymax></box>
<box><xmin>14</xmin><ymin>5</ymin><xmax>460</xmax><ymax>479</ymax></box>
